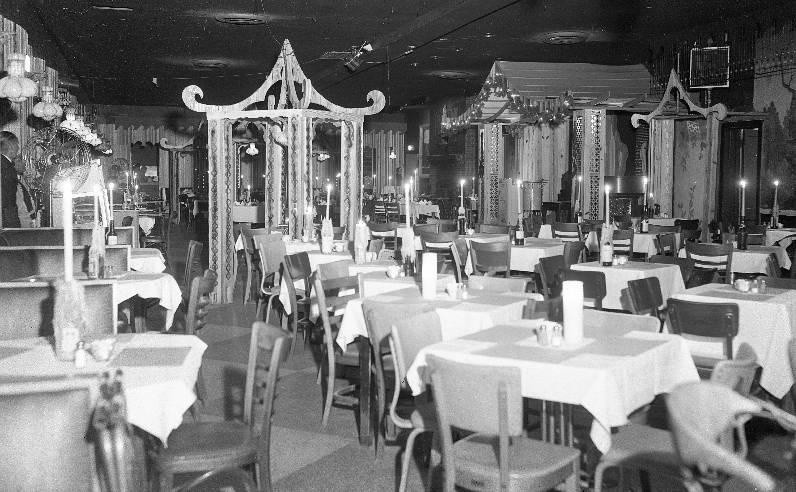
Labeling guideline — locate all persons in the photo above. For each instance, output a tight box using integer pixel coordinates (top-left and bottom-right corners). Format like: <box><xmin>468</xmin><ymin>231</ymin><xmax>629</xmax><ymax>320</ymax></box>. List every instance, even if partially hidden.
<box><xmin>0</xmin><ymin>130</ymin><xmax>21</xmax><ymax>229</ymax></box>
<box><xmin>12</xmin><ymin>149</ymin><xmax>37</xmax><ymax>229</ymax></box>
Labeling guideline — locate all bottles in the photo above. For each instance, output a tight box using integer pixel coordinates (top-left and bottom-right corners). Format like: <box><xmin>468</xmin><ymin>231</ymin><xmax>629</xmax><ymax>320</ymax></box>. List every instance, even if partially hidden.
<box><xmin>404</xmin><ymin>252</ymin><xmax>414</xmax><ymax>276</ymax></box>
<box><xmin>640</xmin><ymin>206</ymin><xmax>649</xmax><ymax>232</ymax></box>
<box><xmin>771</xmin><ymin>212</ymin><xmax>777</xmax><ymax>228</ymax></box>
<box><xmin>107</xmin><ymin>264</ymin><xmax>115</xmax><ymax>276</ymax></box>
<box><xmin>752</xmin><ymin>279</ymin><xmax>768</xmax><ymax>293</ymax></box>
<box><xmin>75</xmin><ymin>340</ymin><xmax>87</xmax><ymax>366</ymax></box>
<box><xmin>537</xmin><ymin>322</ymin><xmax>561</xmax><ymax>348</ymax></box>
<box><xmin>106</xmin><ymin>219</ymin><xmax>118</xmax><ymax>245</ymax></box>
<box><xmin>515</xmin><ymin>217</ymin><xmax>524</xmax><ymax>245</ymax></box>
<box><xmin>455</xmin><ymin>283</ymin><xmax>468</xmax><ymax>300</ymax></box>
<box><xmin>456</xmin><ymin>208</ymin><xmax>466</xmax><ymax>235</ymax></box>
<box><xmin>600</xmin><ymin>223</ymin><xmax>614</xmax><ymax>266</ymax></box>
<box><xmin>87</xmin><ymin>229</ymin><xmax>104</xmax><ymax>280</ymax></box>
<box><xmin>37</xmin><ymin>197</ymin><xmax>47</xmax><ymax>227</ymax></box>
<box><xmin>52</xmin><ymin>280</ymin><xmax>83</xmax><ymax>359</ymax></box>
<box><xmin>739</xmin><ymin>217</ymin><xmax>747</xmax><ymax>249</ymax></box>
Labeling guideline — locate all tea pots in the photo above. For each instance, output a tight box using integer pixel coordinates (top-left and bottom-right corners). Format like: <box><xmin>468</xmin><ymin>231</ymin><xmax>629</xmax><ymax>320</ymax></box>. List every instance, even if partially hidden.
<box><xmin>304</xmin><ymin>229</ymin><xmax>319</xmax><ymax>243</ymax></box>
<box><xmin>331</xmin><ymin>241</ymin><xmax>349</xmax><ymax>252</ymax></box>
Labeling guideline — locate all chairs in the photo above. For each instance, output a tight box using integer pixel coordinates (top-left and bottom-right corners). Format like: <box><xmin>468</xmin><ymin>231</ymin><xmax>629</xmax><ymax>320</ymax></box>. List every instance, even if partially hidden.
<box><xmin>0</xmin><ymin>165</ymin><xmax>795</xmax><ymax>492</ymax></box>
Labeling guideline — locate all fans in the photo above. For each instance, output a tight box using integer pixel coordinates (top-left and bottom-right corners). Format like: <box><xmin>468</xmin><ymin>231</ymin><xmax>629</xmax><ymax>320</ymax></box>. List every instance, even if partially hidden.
<box><xmin>22</xmin><ymin>123</ymin><xmax>90</xmax><ymax>227</ymax></box>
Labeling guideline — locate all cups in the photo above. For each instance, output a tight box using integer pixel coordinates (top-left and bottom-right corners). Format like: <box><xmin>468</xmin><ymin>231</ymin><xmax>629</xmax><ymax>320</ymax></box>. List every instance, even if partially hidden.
<box><xmin>764</xmin><ymin>222</ymin><xmax>783</xmax><ymax>229</ymax></box>
<box><xmin>732</xmin><ymin>240</ymin><xmax>738</xmax><ymax>249</ymax></box>
<box><xmin>509</xmin><ymin>229</ymin><xmax>516</xmax><ymax>245</ymax></box>
<box><xmin>612</xmin><ymin>255</ymin><xmax>629</xmax><ymax>266</ymax></box>
<box><xmin>387</xmin><ymin>265</ymin><xmax>405</xmax><ymax>278</ymax></box>
<box><xmin>90</xmin><ymin>339</ymin><xmax>117</xmax><ymax>363</ymax></box>
<box><xmin>734</xmin><ymin>279</ymin><xmax>750</xmax><ymax>292</ymax></box>
<box><xmin>448</xmin><ymin>283</ymin><xmax>461</xmax><ymax>296</ymax></box>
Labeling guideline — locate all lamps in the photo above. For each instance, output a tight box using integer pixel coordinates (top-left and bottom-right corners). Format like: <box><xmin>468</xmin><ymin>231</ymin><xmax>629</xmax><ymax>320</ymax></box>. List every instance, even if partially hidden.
<box><xmin>0</xmin><ymin>50</ymin><xmax>39</xmax><ymax>103</ymax></box>
<box><xmin>234</xmin><ymin>139</ymin><xmax>398</xmax><ymax>164</ymax></box>
<box><xmin>30</xmin><ymin>85</ymin><xmax>64</xmax><ymax>121</ymax></box>
<box><xmin>56</xmin><ymin>93</ymin><xmax>115</xmax><ymax>155</ymax></box>
